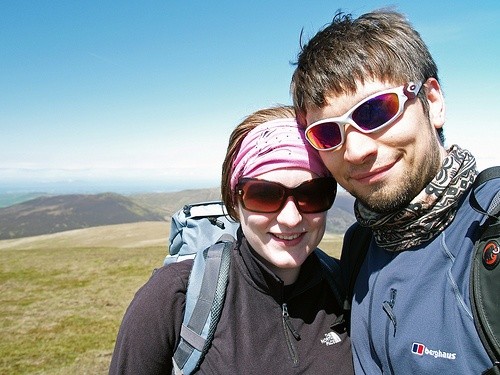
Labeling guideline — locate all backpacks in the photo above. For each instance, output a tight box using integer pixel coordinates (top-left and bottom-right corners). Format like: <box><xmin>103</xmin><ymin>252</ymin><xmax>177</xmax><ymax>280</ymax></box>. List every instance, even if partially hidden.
<box><xmin>164</xmin><ymin>202</ymin><xmax>340</xmax><ymax>375</ymax></box>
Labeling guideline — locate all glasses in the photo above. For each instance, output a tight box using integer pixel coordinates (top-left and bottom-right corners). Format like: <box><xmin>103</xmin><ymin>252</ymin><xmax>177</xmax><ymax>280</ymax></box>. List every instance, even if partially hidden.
<box><xmin>236</xmin><ymin>178</ymin><xmax>337</xmax><ymax>213</ymax></box>
<box><xmin>304</xmin><ymin>80</ymin><xmax>422</xmax><ymax>151</ymax></box>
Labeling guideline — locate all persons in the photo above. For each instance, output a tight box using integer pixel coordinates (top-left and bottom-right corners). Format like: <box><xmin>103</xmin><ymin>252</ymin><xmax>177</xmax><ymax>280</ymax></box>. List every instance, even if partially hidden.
<box><xmin>287</xmin><ymin>5</ymin><xmax>499</xmax><ymax>375</ymax></box>
<box><xmin>103</xmin><ymin>101</ymin><xmax>375</xmax><ymax>374</ymax></box>
